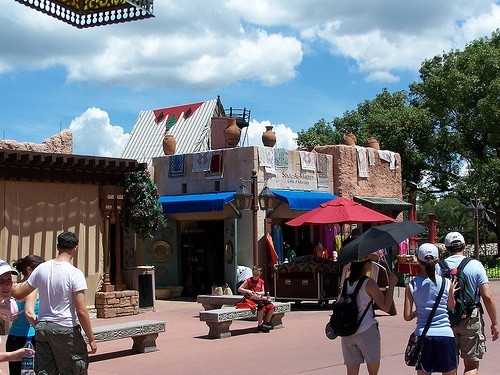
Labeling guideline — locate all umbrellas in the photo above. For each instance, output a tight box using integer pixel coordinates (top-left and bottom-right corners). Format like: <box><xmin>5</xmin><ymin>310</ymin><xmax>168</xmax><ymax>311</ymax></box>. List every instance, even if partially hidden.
<box><xmin>284</xmin><ymin>198</ymin><xmax>396</xmax><ymax>249</ymax></box>
<box><xmin>336</xmin><ymin>220</ymin><xmax>429</xmax><ymax>274</ymax></box>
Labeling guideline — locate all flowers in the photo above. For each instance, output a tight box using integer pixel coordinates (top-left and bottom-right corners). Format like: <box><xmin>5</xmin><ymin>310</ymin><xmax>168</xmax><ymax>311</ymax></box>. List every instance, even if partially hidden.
<box><xmin>120</xmin><ymin>171</ymin><xmax>168</xmax><ymax>243</ymax></box>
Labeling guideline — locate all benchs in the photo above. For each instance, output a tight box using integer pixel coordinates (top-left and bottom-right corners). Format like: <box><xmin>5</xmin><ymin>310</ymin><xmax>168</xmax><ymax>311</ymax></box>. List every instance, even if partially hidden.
<box><xmin>197</xmin><ymin>295</ymin><xmax>275</xmax><ymax>311</ymax></box>
<box><xmin>80</xmin><ymin>320</ymin><xmax>166</xmax><ymax>354</ymax></box>
<box><xmin>199</xmin><ymin>302</ymin><xmax>290</xmax><ymax>338</ymax></box>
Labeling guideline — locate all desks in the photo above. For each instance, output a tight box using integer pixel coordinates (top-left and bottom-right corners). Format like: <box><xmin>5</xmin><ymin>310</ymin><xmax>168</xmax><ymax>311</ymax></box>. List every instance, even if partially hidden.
<box><xmin>393</xmin><ymin>261</ymin><xmax>423</xmax><ymax>282</ymax></box>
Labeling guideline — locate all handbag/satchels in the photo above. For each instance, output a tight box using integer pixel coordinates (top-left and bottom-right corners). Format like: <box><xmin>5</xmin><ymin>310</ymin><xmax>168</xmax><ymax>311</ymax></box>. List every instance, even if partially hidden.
<box><xmin>404</xmin><ymin>332</ymin><xmax>425</xmax><ymax>366</ymax></box>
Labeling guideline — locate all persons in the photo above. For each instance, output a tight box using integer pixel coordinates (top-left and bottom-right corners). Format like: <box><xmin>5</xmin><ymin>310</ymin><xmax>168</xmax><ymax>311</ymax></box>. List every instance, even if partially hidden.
<box><xmin>11</xmin><ymin>231</ymin><xmax>97</xmax><ymax>375</ymax></box>
<box><xmin>443</xmin><ymin>232</ymin><xmax>500</xmax><ymax>375</ymax></box>
<box><xmin>404</xmin><ymin>243</ymin><xmax>461</xmax><ymax>375</ymax></box>
<box><xmin>238</xmin><ymin>264</ymin><xmax>274</xmax><ymax>333</ymax></box>
<box><xmin>0</xmin><ymin>254</ymin><xmax>46</xmax><ymax>375</ymax></box>
<box><xmin>342</xmin><ymin>228</ymin><xmax>361</xmax><ymax>248</ymax></box>
<box><xmin>337</xmin><ymin>253</ymin><xmax>398</xmax><ymax>375</ymax></box>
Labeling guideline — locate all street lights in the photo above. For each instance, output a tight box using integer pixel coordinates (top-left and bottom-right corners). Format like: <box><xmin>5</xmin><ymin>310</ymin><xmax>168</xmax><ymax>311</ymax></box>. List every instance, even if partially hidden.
<box><xmin>235</xmin><ymin>168</ymin><xmax>278</xmax><ymax>271</ymax></box>
<box><xmin>466</xmin><ymin>187</ymin><xmax>486</xmax><ymax>260</ymax></box>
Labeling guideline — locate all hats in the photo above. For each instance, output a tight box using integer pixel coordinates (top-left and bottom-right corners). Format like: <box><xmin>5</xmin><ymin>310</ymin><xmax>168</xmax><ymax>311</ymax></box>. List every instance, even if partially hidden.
<box><xmin>417</xmin><ymin>243</ymin><xmax>439</xmax><ymax>263</ymax></box>
<box><xmin>351</xmin><ymin>253</ymin><xmax>379</xmax><ymax>262</ymax></box>
<box><xmin>324</xmin><ymin>321</ymin><xmax>338</xmax><ymax>339</ymax></box>
<box><xmin>444</xmin><ymin>232</ymin><xmax>464</xmax><ymax>248</ymax></box>
<box><xmin>0</xmin><ymin>258</ymin><xmax>19</xmax><ymax>276</ymax></box>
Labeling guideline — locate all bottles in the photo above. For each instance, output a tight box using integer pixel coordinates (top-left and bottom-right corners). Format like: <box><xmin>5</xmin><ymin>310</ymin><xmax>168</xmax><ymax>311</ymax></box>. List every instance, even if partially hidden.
<box><xmin>267</xmin><ymin>292</ymin><xmax>271</xmax><ymax>302</ymax></box>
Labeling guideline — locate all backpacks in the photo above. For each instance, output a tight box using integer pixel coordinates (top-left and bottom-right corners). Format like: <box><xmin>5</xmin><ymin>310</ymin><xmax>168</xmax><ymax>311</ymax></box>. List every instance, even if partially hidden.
<box><xmin>329</xmin><ymin>275</ymin><xmax>373</xmax><ymax>337</ymax></box>
<box><xmin>438</xmin><ymin>256</ymin><xmax>475</xmax><ymax>326</ymax></box>
<box><xmin>0</xmin><ymin>283</ymin><xmax>26</xmax><ymax>322</ymax></box>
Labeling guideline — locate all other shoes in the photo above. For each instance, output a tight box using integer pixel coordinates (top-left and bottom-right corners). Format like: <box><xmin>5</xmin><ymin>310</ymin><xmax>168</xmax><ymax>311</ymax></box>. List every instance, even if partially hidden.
<box><xmin>256</xmin><ymin>322</ymin><xmax>273</xmax><ymax>333</ymax></box>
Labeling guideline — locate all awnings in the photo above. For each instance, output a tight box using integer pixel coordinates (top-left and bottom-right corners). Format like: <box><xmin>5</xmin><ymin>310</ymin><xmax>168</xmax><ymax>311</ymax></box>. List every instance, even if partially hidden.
<box><xmin>266</xmin><ymin>189</ymin><xmax>338</xmax><ymax>219</ymax></box>
<box><xmin>354</xmin><ymin>196</ymin><xmax>413</xmax><ymax>220</ymax></box>
<box><xmin>159</xmin><ymin>191</ymin><xmax>242</xmax><ymax>219</ymax></box>
